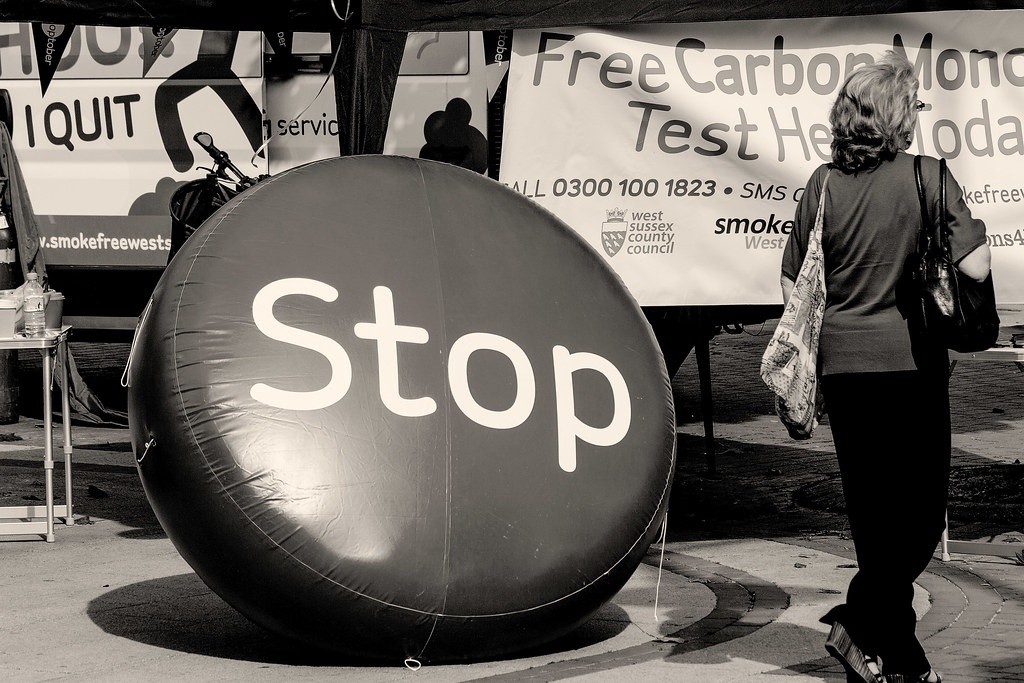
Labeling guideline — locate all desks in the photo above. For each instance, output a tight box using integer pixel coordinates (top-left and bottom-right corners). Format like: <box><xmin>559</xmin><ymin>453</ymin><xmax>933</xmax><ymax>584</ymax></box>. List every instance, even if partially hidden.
<box><xmin>940</xmin><ymin>344</ymin><xmax>1024</xmax><ymax>561</ymax></box>
<box><xmin>1</xmin><ymin>324</ymin><xmax>73</xmax><ymax>542</ymax></box>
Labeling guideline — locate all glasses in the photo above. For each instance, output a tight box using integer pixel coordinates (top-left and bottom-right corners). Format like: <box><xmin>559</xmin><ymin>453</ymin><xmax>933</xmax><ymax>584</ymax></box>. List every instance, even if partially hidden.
<box><xmin>915</xmin><ymin>99</ymin><xmax>925</xmax><ymax>112</ymax></box>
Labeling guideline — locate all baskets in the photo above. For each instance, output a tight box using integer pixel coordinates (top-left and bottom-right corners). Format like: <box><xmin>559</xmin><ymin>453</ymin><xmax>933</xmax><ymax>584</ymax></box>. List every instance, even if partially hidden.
<box><xmin>167</xmin><ymin>178</ymin><xmax>239</xmax><ymax>267</ymax></box>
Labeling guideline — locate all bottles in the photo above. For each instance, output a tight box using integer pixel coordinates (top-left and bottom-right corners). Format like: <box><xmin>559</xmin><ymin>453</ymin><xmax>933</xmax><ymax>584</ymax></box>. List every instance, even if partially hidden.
<box><xmin>23</xmin><ymin>272</ymin><xmax>46</xmax><ymax>339</ymax></box>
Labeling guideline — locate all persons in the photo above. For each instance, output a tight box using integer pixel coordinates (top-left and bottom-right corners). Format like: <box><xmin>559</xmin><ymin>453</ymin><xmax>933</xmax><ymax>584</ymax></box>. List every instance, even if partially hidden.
<box><xmin>781</xmin><ymin>51</ymin><xmax>991</xmax><ymax>683</ymax></box>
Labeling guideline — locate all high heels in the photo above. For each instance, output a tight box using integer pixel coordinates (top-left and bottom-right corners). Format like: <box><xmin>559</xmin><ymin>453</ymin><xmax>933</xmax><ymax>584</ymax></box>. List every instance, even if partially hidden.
<box><xmin>888</xmin><ymin>668</ymin><xmax>942</xmax><ymax>683</ymax></box>
<box><xmin>825</xmin><ymin>621</ymin><xmax>886</xmax><ymax>683</ymax></box>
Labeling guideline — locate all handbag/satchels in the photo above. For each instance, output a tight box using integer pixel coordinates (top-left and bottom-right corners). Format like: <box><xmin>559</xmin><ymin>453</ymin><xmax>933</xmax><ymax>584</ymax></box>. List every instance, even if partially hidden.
<box><xmin>911</xmin><ymin>155</ymin><xmax>1000</xmax><ymax>352</ymax></box>
<box><xmin>759</xmin><ymin>163</ymin><xmax>831</xmax><ymax>440</ymax></box>
